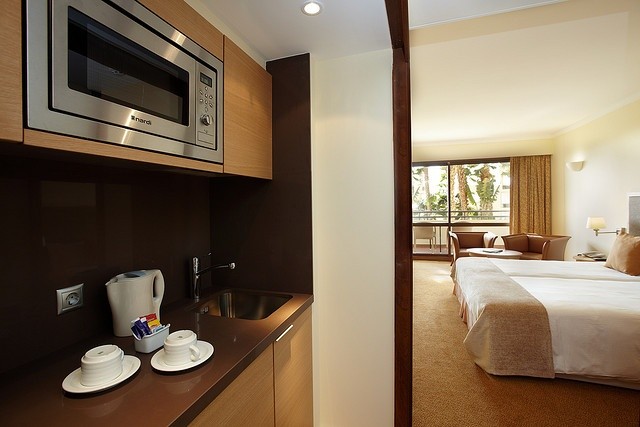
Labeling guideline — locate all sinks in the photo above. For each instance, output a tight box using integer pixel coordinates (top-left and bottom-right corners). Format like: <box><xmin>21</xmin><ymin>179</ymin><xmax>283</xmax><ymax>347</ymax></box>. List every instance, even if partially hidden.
<box><xmin>198</xmin><ymin>290</ymin><xmax>292</xmax><ymax>319</ymax></box>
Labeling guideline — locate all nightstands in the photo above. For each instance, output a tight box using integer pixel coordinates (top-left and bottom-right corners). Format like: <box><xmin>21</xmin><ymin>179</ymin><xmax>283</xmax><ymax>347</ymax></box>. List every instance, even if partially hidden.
<box><xmin>573</xmin><ymin>253</ymin><xmax>608</xmax><ymax>263</ymax></box>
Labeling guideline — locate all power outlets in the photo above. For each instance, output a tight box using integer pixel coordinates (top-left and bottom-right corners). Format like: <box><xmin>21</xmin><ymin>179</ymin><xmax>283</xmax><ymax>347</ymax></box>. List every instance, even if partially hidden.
<box><xmin>56</xmin><ymin>283</ymin><xmax>84</xmax><ymax>314</ymax></box>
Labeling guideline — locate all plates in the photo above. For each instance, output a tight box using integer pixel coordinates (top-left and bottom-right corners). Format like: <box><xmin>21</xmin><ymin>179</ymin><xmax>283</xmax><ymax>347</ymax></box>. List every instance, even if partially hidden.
<box><xmin>61</xmin><ymin>354</ymin><xmax>141</xmax><ymax>395</ymax></box>
<box><xmin>150</xmin><ymin>340</ymin><xmax>214</xmax><ymax>373</ymax></box>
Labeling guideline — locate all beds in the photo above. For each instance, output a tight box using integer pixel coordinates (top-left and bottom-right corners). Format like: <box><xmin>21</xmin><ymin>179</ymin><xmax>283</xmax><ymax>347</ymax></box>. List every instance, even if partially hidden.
<box><xmin>450</xmin><ymin>192</ymin><xmax>639</xmax><ymax>390</ymax></box>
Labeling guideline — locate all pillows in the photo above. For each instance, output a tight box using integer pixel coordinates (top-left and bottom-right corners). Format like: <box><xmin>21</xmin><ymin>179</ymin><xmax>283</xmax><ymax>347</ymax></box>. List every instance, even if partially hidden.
<box><xmin>603</xmin><ymin>233</ymin><xmax>640</xmax><ymax>275</ymax></box>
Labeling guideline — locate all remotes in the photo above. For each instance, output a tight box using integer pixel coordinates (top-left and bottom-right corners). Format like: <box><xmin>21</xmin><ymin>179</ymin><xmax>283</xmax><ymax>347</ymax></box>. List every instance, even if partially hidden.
<box><xmin>594</xmin><ymin>258</ymin><xmax>607</xmax><ymax>261</ymax></box>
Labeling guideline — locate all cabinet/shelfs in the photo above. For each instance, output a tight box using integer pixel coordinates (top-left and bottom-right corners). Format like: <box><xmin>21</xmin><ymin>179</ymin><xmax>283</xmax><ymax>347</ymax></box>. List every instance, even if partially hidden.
<box><xmin>223</xmin><ymin>34</ymin><xmax>272</xmax><ymax>180</ymax></box>
<box><xmin>274</xmin><ymin>304</ymin><xmax>313</xmax><ymax>426</ymax></box>
<box><xmin>186</xmin><ymin>343</ymin><xmax>274</xmax><ymax>427</ymax></box>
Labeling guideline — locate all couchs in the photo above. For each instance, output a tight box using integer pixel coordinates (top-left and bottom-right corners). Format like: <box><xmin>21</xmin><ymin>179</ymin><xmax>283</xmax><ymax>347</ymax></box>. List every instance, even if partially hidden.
<box><xmin>501</xmin><ymin>233</ymin><xmax>572</xmax><ymax>260</ymax></box>
<box><xmin>449</xmin><ymin>230</ymin><xmax>498</xmax><ymax>266</ymax></box>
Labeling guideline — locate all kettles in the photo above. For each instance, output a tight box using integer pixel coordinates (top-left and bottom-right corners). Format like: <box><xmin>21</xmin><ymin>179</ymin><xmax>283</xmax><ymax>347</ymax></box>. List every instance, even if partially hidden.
<box><xmin>104</xmin><ymin>269</ymin><xmax>166</xmax><ymax>338</ymax></box>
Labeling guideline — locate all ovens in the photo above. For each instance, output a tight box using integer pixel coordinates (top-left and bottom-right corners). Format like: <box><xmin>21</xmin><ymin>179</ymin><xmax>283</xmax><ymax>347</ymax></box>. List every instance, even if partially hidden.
<box><xmin>23</xmin><ymin>1</ymin><xmax>223</xmax><ymax>164</ymax></box>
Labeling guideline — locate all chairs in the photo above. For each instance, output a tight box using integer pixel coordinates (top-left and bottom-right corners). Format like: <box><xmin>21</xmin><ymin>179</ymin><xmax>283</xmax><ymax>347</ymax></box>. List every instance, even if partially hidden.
<box><xmin>414</xmin><ymin>225</ymin><xmax>436</xmax><ymax>251</ymax></box>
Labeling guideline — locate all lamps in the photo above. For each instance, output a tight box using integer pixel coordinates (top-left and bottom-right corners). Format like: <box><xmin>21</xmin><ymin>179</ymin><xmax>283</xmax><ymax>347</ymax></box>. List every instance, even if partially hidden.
<box><xmin>565</xmin><ymin>159</ymin><xmax>584</xmax><ymax>172</ymax></box>
<box><xmin>586</xmin><ymin>216</ymin><xmax>626</xmax><ymax>235</ymax></box>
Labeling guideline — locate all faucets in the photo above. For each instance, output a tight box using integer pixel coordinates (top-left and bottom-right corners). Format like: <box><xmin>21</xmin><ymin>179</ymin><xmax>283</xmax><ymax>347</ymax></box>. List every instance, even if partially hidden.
<box><xmin>192</xmin><ymin>252</ymin><xmax>236</xmax><ymax>301</ymax></box>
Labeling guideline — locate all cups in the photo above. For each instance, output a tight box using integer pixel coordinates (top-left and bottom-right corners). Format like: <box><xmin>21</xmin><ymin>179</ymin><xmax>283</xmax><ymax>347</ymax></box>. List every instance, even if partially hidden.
<box><xmin>163</xmin><ymin>329</ymin><xmax>201</xmax><ymax>366</ymax></box>
<box><xmin>80</xmin><ymin>344</ymin><xmax>125</xmax><ymax>387</ymax></box>
